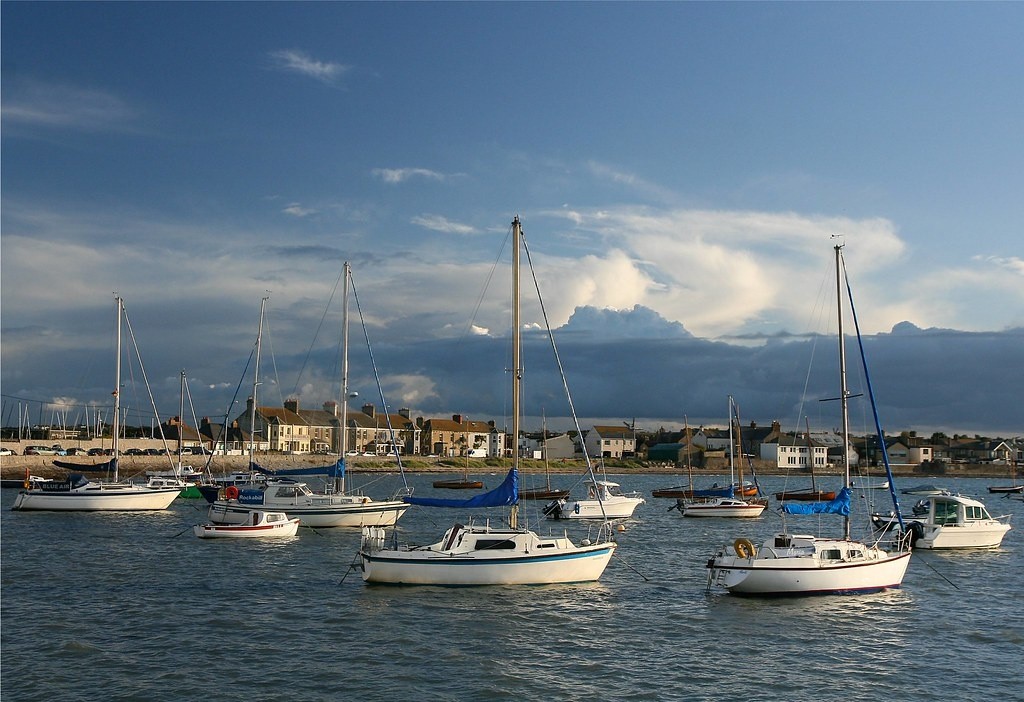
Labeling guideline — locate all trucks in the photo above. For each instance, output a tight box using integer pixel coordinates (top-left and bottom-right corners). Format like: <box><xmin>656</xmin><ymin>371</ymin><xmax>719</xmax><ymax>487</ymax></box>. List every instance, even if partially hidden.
<box><xmin>466</xmin><ymin>449</ymin><xmax>487</xmax><ymax>459</ymax></box>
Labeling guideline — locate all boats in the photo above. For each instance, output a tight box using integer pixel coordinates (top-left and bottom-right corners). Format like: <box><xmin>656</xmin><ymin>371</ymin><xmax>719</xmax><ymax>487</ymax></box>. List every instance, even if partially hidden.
<box><xmin>777</xmin><ymin>413</ymin><xmax>835</xmax><ymax>501</ymax></box>
<box><xmin>666</xmin><ymin>395</ymin><xmax>768</xmax><ymax>521</ymax></box>
<box><xmin>849</xmin><ymin>480</ymin><xmax>890</xmax><ymax>488</ymax></box>
<box><xmin>143</xmin><ymin>465</ymin><xmax>203</xmax><ymax>478</ymax></box>
<box><xmin>541</xmin><ymin>451</ymin><xmax>645</xmax><ymax>519</ymax></box>
<box><xmin>0</xmin><ymin>472</ymin><xmax>54</xmax><ymax>489</ymax></box>
<box><xmin>989</xmin><ymin>485</ymin><xmax>1024</xmax><ymax>493</ymax></box>
<box><xmin>903</xmin><ymin>484</ymin><xmax>946</xmax><ymax>496</ymax></box>
<box><xmin>896</xmin><ymin>492</ymin><xmax>1014</xmax><ymax>548</ymax></box>
<box><xmin>870</xmin><ymin>498</ymin><xmax>961</xmax><ymax>534</ymax></box>
<box><xmin>197</xmin><ymin>510</ymin><xmax>303</xmax><ymax>542</ymax></box>
<box><xmin>652</xmin><ymin>405</ymin><xmax>761</xmax><ymax>498</ymax></box>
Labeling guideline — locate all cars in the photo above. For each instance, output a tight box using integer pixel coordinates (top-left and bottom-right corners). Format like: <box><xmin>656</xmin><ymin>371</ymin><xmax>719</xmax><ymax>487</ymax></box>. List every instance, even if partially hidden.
<box><xmin>0</xmin><ymin>433</ymin><xmax>213</xmax><ymax>457</ymax></box>
<box><xmin>258</xmin><ymin>444</ymin><xmax>441</xmax><ymax>459</ymax></box>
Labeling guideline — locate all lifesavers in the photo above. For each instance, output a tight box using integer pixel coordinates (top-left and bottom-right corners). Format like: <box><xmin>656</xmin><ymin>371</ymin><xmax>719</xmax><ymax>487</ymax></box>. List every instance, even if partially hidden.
<box><xmin>24</xmin><ymin>480</ymin><xmax>30</xmax><ymax>489</ymax></box>
<box><xmin>734</xmin><ymin>538</ymin><xmax>755</xmax><ymax>559</ymax></box>
<box><xmin>225</xmin><ymin>486</ymin><xmax>238</xmax><ymax>499</ymax></box>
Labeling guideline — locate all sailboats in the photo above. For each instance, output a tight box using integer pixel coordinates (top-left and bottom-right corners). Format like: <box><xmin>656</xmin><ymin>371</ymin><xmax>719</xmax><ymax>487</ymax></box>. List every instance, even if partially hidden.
<box><xmin>432</xmin><ymin>419</ymin><xmax>482</xmax><ymax>489</ymax></box>
<box><xmin>10</xmin><ymin>296</ymin><xmax>183</xmax><ymax>511</ymax></box>
<box><xmin>143</xmin><ymin>368</ymin><xmax>267</xmax><ymax>490</ymax></box>
<box><xmin>192</xmin><ymin>294</ymin><xmax>301</xmax><ymax>502</ymax></box>
<box><xmin>193</xmin><ymin>261</ymin><xmax>417</xmax><ymax>529</ymax></box>
<box><xmin>361</xmin><ymin>208</ymin><xmax>617</xmax><ymax>596</ymax></box>
<box><xmin>515</xmin><ymin>406</ymin><xmax>572</xmax><ymax>501</ymax></box>
<box><xmin>703</xmin><ymin>248</ymin><xmax>911</xmax><ymax>601</ymax></box>
